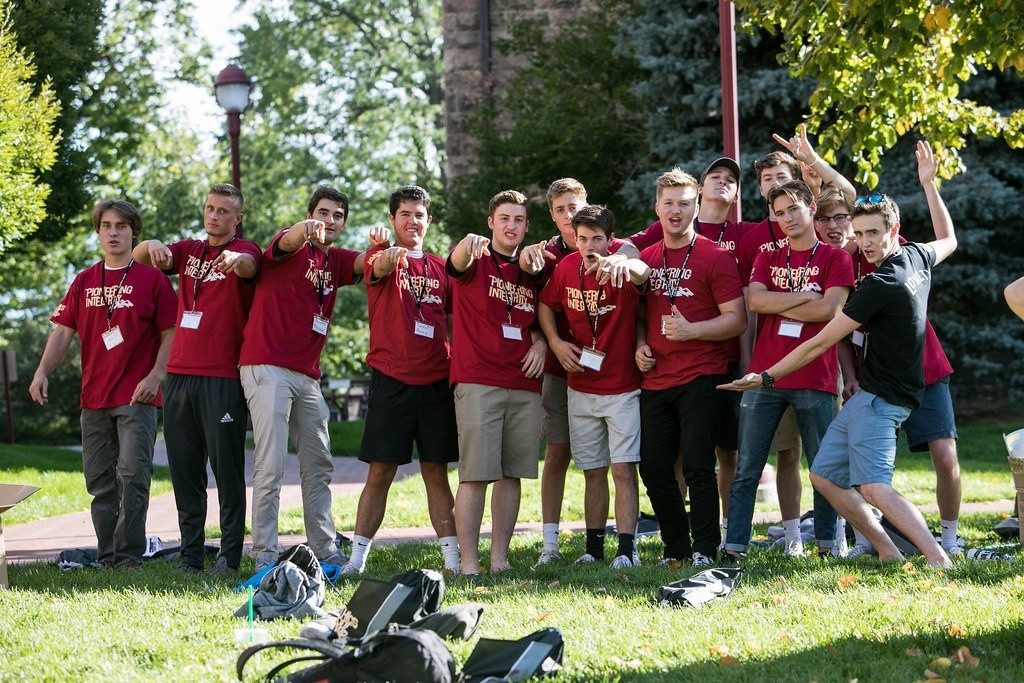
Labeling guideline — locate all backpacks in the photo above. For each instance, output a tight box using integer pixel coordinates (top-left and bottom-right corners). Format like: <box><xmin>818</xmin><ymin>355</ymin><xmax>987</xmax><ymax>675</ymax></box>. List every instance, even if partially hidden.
<box><xmin>389</xmin><ymin>570</ymin><xmax>445</xmax><ymax>628</ymax></box>
<box><xmin>238</xmin><ymin>629</ymin><xmax>456</xmax><ymax>683</ymax></box>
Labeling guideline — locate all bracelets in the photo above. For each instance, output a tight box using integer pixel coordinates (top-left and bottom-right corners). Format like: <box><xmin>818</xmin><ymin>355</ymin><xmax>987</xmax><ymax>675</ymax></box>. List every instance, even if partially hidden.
<box><xmin>806</xmin><ymin>153</ymin><xmax>818</xmax><ymax>166</ymax></box>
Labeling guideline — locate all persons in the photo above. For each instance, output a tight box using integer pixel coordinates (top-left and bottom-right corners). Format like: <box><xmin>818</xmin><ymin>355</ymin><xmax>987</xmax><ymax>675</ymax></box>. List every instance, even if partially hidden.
<box><xmin>635</xmin><ymin>167</ymin><xmax>748</xmax><ymax>572</ymax></box>
<box><xmin>716</xmin><ymin>140</ymin><xmax>958</xmax><ymax>574</ymax></box>
<box><xmin>241</xmin><ymin>186</ymin><xmax>390</xmax><ymax>582</ymax></box>
<box><xmin>519</xmin><ymin>178</ymin><xmax>641</xmax><ymax>570</ymax></box>
<box><xmin>737</xmin><ymin>123</ymin><xmax>857</xmax><ymax>558</ymax></box>
<box><xmin>131</xmin><ymin>183</ymin><xmax>262</xmax><ymax>579</ymax></box>
<box><xmin>340</xmin><ymin>187</ymin><xmax>461</xmax><ymax>577</ymax></box>
<box><xmin>539</xmin><ymin>206</ymin><xmax>652</xmax><ymax>570</ymax></box>
<box><xmin>719</xmin><ymin>179</ymin><xmax>854</xmax><ymax>565</ymax></box>
<box><xmin>29</xmin><ymin>201</ymin><xmax>179</xmax><ymax>571</ymax></box>
<box><xmin>811</xmin><ymin>187</ymin><xmax>968</xmax><ymax>557</ymax></box>
<box><xmin>445</xmin><ymin>191</ymin><xmax>549</xmax><ymax>579</ymax></box>
<box><xmin>547</xmin><ymin>156</ymin><xmax>826</xmax><ymax>552</ymax></box>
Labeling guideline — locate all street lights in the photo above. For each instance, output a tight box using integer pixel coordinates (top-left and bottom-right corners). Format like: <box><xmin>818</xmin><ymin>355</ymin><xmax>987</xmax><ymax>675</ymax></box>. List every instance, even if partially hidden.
<box><xmin>214</xmin><ymin>62</ymin><xmax>253</xmax><ymax>240</ymax></box>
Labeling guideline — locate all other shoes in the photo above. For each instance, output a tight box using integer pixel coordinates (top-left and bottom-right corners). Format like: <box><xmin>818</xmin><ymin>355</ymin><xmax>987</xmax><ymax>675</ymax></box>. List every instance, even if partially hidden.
<box><xmin>610</xmin><ymin>555</ymin><xmax>641</xmax><ymax>570</ymax></box>
<box><xmin>442</xmin><ymin>563</ymin><xmax>460</xmax><ymax>580</ymax></box>
<box><xmin>338</xmin><ymin>561</ymin><xmax>363</xmax><ymax>575</ymax></box>
<box><xmin>720</xmin><ymin>548</ymin><xmax>740</xmax><ymax>565</ymax></box>
<box><xmin>574</xmin><ymin>554</ymin><xmax>595</xmax><ymax>565</ymax></box>
<box><xmin>653</xmin><ymin>558</ymin><xmax>684</xmax><ymax>570</ymax></box>
<box><xmin>848</xmin><ymin>543</ymin><xmax>873</xmax><ymax>557</ymax></box>
<box><xmin>534</xmin><ymin>549</ymin><xmax>563</xmax><ymax>566</ymax></box>
<box><xmin>176</xmin><ymin>563</ymin><xmax>200</xmax><ymax>574</ymax></box>
<box><xmin>207</xmin><ymin>557</ymin><xmax>237</xmax><ymax>579</ymax></box>
<box><xmin>251</xmin><ymin>558</ymin><xmax>271</xmax><ymax>574</ymax></box>
<box><xmin>832</xmin><ymin>533</ymin><xmax>849</xmax><ymax>557</ymax></box>
<box><xmin>691</xmin><ymin>551</ymin><xmax>715</xmax><ymax>566</ymax></box>
<box><xmin>317</xmin><ymin>550</ymin><xmax>349</xmax><ymax>566</ymax></box>
<box><xmin>780</xmin><ymin>534</ymin><xmax>803</xmax><ymax>559</ymax></box>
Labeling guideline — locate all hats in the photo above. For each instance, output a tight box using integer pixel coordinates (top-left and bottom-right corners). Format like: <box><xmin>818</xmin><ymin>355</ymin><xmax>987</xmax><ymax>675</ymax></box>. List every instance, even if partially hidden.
<box><xmin>699</xmin><ymin>157</ymin><xmax>740</xmax><ymax>183</ymax></box>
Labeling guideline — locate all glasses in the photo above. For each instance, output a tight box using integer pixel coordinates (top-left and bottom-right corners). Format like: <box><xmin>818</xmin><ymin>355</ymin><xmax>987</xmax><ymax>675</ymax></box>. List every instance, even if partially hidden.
<box><xmin>853</xmin><ymin>195</ymin><xmax>895</xmax><ymax>212</ymax></box>
<box><xmin>815</xmin><ymin>214</ymin><xmax>850</xmax><ymax>225</ymax></box>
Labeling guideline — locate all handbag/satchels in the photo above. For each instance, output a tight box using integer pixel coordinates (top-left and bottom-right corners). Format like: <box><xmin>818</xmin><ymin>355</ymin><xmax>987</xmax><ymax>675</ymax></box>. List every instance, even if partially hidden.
<box><xmin>407</xmin><ymin>604</ymin><xmax>486</xmax><ymax>641</ymax></box>
<box><xmin>329</xmin><ymin>579</ymin><xmax>413</xmax><ymax>645</ymax></box>
<box><xmin>233</xmin><ymin>544</ymin><xmax>326</xmax><ymax>620</ymax></box>
<box><xmin>462</xmin><ymin>627</ymin><xmax>563</xmax><ymax>683</ymax></box>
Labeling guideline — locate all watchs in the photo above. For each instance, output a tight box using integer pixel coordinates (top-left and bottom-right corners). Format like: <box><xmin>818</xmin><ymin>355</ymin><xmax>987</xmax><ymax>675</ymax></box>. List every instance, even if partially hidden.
<box><xmin>761</xmin><ymin>372</ymin><xmax>775</xmax><ymax>387</ymax></box>
